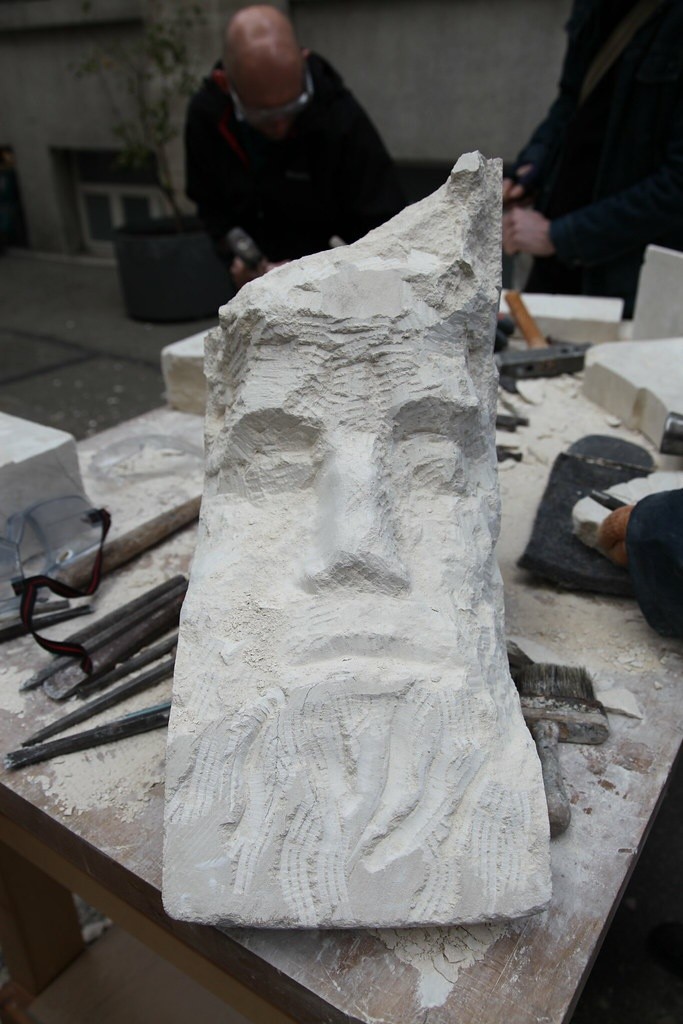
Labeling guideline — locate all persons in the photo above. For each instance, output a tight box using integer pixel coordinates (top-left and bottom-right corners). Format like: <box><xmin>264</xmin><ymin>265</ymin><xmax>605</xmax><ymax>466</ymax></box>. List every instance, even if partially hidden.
<box><xmin>184</xmin><ymin>6</ymin><xmax>402</xmax><ymax>290</ymax></box>
<box><xmin>502</xmin><ymin>0</ymin><xmax>683</xmax><ymax>321</ymax></box>
<box><xmin>600</xmin><ymin>489</ymin><xmax>683</xmax><ymax>639</ymax></box>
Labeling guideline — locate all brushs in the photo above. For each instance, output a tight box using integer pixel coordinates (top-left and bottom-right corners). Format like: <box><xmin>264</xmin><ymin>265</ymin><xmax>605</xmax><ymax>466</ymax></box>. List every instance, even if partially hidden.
<box><xmin>516</xmin><ymin>662</ymin><xmax>611</xmax><ymax>836</ymax></box>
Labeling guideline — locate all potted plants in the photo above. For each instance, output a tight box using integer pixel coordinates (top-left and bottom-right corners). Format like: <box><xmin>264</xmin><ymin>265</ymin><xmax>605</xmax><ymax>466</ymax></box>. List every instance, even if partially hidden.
<box><xmin>62</xmin><ymin>0</ymin><xmax>240</xmax><ymax>323</ymax></box>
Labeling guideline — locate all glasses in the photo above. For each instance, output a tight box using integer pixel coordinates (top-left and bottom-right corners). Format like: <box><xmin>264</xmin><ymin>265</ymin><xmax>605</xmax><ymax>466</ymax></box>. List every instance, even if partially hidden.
<box><xmin>230</xmin><ymin>72</ymin><xmax>315</xmax><ymax>122</ymax></box>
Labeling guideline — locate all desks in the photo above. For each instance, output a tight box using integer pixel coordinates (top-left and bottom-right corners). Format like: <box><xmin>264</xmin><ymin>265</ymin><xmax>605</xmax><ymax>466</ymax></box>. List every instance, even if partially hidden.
<box><xmin>1</xmin><ymin>372</ymin><xmax>683</xmax><ymax>1024</ymax></box>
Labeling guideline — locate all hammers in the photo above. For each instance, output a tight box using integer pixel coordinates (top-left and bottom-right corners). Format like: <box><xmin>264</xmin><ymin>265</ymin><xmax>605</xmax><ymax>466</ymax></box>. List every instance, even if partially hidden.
<box><xmin>497</xmin><ymin>291</ymin><xmax>592</xmax><ymax>380</ymax></box>
<box><xmin>227</xmin><ymin>226</ymin><xmax>262</xmax><ymax>273</ymax></box>
